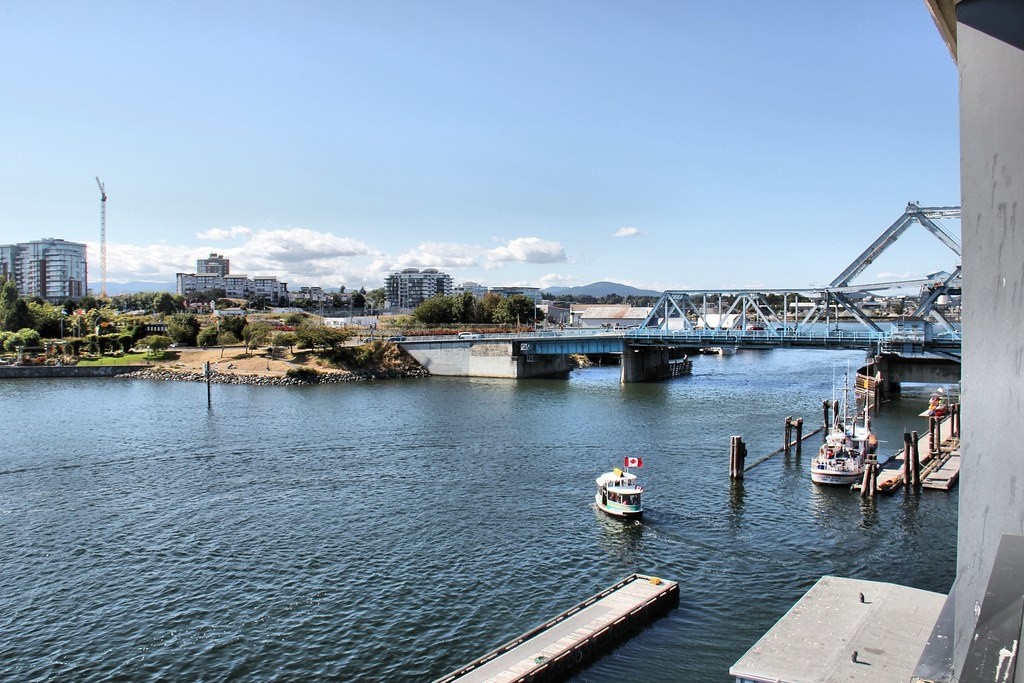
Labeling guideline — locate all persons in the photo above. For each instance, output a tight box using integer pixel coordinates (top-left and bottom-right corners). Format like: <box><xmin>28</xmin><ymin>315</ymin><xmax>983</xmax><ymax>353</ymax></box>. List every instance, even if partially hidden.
<box><xmin>610</xmin><ymin>493</ymin><xmax>640</xmax><ymax>505</ymax></box>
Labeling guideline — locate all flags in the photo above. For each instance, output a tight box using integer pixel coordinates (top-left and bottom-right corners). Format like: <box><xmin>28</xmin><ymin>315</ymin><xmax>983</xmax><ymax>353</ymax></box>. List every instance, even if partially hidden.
<box><xmin>624</xmin><ymin>456</ymin><xmax>643</xmax><ymax>468</ymax></box>
<box><xmin>62</xmin><ymin>308</ymin><xmax>86</xmax><ymax>315</ymax></box>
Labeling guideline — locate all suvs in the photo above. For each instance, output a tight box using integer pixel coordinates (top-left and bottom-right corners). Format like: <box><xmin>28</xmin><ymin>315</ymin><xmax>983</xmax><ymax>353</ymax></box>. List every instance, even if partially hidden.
<box><xmin>459</xmin><ymin>332</ymin><xmax>486</xmax><ymax>340</ymax></box>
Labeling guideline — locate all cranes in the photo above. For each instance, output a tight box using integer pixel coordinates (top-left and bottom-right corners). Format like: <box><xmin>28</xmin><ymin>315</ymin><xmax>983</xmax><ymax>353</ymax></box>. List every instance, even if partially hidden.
<box><xmin>95</xmin><ymin>176</ymin><xmax>108</xmax><ymax>299</ymax></box>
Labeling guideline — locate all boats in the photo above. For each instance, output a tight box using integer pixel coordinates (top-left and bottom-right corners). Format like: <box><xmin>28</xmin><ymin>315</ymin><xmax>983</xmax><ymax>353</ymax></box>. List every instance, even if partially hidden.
<box><xmin>810</xmin><ymin>358</ymin><xmax>870</xmax><ymax>489</ymax></box>
<box><xmin>594</xmin><ymin>466</ymin><xmax>644</xmax><ymax>520</ymax></box>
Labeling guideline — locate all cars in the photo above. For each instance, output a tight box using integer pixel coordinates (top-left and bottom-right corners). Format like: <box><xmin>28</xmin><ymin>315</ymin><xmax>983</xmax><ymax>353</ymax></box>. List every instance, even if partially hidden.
<box><xmin>363</xmin><ymin>337</ymin><xmax>379</xmax><ymax>344</ymax></box>
<box><xmin>388</xmin><ymin>334</ymin><xmax>406</xmax><ymax>341</ymax></box>
